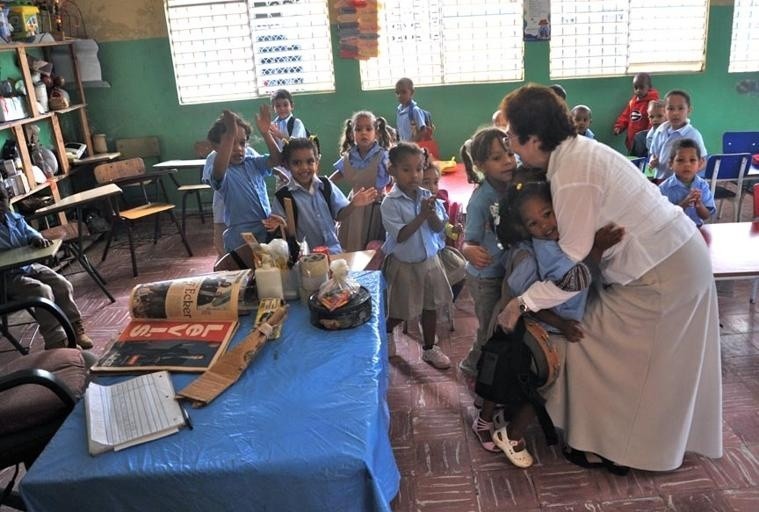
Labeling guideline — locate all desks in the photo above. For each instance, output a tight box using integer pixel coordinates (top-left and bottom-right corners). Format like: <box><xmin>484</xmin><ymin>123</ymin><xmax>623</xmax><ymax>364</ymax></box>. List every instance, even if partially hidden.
<box><xmin>17</xmin><ymin>270</ymin><xmax>401</xmax><ymax>512</ymax></box>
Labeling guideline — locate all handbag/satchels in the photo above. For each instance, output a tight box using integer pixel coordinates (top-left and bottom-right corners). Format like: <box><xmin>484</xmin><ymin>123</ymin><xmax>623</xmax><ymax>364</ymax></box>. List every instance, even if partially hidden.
<box><xmin>475</xmin><ymin>316</ymin><xmax>532</xmax><ymax>404</ymax></box>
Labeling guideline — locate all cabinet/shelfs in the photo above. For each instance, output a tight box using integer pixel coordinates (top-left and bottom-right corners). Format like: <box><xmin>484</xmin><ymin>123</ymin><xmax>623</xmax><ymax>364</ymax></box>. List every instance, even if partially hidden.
<box><xmin>0</xmin><ymin>38</ymin><xmax>108</xmax><ymax>274</ymax></box>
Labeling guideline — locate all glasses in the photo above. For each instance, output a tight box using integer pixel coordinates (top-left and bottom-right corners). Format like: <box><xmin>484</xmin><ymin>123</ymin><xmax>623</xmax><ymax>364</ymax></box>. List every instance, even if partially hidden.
<box><xmin>503</xmin><ymin>136</ymin><xmax>518</xmax><ymax>150</ymax></box>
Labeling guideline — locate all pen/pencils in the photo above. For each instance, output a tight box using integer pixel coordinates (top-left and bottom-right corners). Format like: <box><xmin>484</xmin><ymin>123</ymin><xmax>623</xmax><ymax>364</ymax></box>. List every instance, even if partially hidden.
<box><xmin>178</xmin><ymin>399</ymin><xmax>195</xmax><ymax>430</ymax></box>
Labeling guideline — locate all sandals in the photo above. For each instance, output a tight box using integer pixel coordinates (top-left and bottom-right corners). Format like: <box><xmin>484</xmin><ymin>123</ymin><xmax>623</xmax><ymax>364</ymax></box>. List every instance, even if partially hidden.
<box><xmin>471</xmin><ymin>410</ymin><xmax>534</xmax><ymax>468</ymax></box>
<box><xmin>563</xmin><ymin>446</ymin><xmax>630</xmax><ymax>476</ymax></box>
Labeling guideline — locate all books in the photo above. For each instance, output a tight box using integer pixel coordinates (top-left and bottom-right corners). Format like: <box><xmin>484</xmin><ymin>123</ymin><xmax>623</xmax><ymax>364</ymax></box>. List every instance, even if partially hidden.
<box><xmin>88</xmin><ymin>265</ymin><xmax>255</xmax><ymax>376</ymax></box>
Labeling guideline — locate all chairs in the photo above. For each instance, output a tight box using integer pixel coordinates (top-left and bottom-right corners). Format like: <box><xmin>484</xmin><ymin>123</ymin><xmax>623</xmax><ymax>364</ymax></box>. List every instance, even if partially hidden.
<box><xmin>624</xmin><ymin>132</ymin><xmax>759</xmax><ymax>306</ymax></box>
<box><xmin>0</xmin><ymin>297</ymin><xmax>84</xmax><ymax>505</ymax></box>
<box><xmin>0</xmin><ymin>238</ymin><xmax>116</xmax><ymax>355</ymax></box>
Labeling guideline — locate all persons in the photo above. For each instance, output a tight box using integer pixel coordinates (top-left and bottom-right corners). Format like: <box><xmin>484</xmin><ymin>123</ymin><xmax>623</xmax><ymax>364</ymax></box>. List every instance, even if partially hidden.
<box><xmin>269</xmin><ymin>89</ymin><xmax>307</xmax><ymax>191</ymax></box>
<box><xmin>0</xmin><ymin>181</ymin><xmax>94</xmax><ymax>351</ymax></box>
<box><xmin>392</xmin><ymin>78</ymin><xmax>427</xmax><ymax>143</ymax></box>
<box><xmin>202</xmin><ymin>146</ymin><xmax>261</xmax><ymax>259</ymax></box>
<box><xmin>123</xmin><ymin>272</ymin><xmax>232</xmax><ymax>367</ymax></box>
<box><xmin>261</xmin><ymin>138</ymin><xmax>378</xmax><ymax>255</ymax></box>
<box><xmin>421</xmin><ymin>162</ymin><xmax>468</xmax><ymax>303</ymax></box>
<box><xmin>381</xmin><ymin>140</ymin><xmax>452</xmax><ymax>370</ymax></box>
<box><xmin>457</xmin><ymin>72</ymin><xmax>723</xmax><ymax>477</ymax></box>
<box><xmin>202</xmin><ymin>103</ymin><xmax>282</xmax><ymax>252</ymax></box>
<box><xmin>327</xmin><ymin>110</ymin><xmax>393</xmax><ymax>254</ymax></box>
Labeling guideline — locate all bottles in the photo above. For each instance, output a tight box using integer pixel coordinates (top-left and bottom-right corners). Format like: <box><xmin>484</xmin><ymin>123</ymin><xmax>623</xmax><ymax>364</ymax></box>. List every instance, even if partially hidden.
<box><xmin>254</xmin><ymin>264</ymin><xmax>285</xmax><ymax>308</ymax></box>
<box><xmin>92</xmin><ymin>133</ymin><xmax>108</xmax><ymax>155</ymax></box>
<box><xmin>312</xmin><ymin>246</ymin><xmax>332</xmax><ymax>275</ymax></box>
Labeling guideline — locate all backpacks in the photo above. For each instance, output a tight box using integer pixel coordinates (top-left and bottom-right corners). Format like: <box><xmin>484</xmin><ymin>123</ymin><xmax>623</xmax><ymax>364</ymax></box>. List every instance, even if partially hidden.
<box><xmin>408</xmin><ymin>104</ymin><xmax>433</xmax><ymax>136</ymax></box>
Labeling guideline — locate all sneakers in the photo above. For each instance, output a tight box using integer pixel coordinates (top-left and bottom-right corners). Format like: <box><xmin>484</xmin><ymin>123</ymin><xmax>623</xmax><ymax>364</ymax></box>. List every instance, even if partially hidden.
<box><xmin>422</xmin><ymin>346</ymin><xmax>451</xmax><ymax>369</ymax></box>
<box><xmin>72</xmin><ymin>319</ymin><xmax>94</xmax><ymax>348</ymax></box>
<box><xmin>44</xmin><ymin>337</ymin><xmax>83</xmax><ymax>351</ymax></box>
<box><xmin>387</xmin><ymin>332</ymin><xmax>396</xmax><ymax>357</ymax></box>
